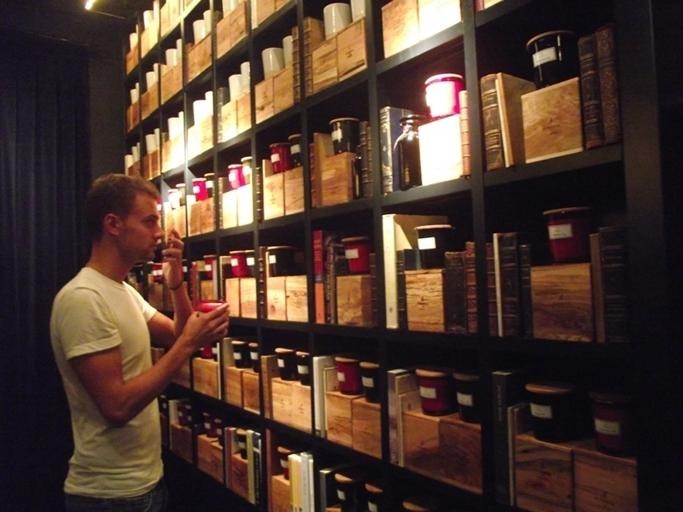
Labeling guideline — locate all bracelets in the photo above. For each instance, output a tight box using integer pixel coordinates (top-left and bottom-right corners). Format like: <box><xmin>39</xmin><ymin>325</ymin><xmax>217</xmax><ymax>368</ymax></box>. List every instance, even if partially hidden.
<box><xmin>167</xmin><ymin>278</ymin><xmax>184</xmax><ymax>290</ymax></box>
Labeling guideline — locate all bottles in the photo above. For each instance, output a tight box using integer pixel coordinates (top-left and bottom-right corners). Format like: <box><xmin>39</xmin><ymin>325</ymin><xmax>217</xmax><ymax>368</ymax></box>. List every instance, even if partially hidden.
<box><xmin>393</xmin><ymin>114</ymin><xmax>423</xmax><ymax>191</ymax></box>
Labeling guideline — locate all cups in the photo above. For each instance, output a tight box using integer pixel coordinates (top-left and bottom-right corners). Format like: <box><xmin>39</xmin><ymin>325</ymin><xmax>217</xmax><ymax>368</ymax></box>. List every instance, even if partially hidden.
<box><xmin>129</xmin><ymin>1</ymin><xmax>238</xmax><ymax>93</ymax></box>
<box><xmin>227</xmin><ymin>62</ymin><xmax>249</xmax><ymax>102</ymax></box>
<box><xmin>123</xmin><ymin>89</ymin><xmax>213</xmax><ymax>175</ymax></box>
<box><xmin>262</xmin><ymin>47</ymin><xmax>285</xmax><ymax>79</ymax></box>
<box><xmin>320</xmin><ymin>2</ymin><xmax>350</xmax><ymax>40</ymax></box>
<box><xmin>280</xmin><ymin>36</ymin><xmax>297</xmax><ymax>64</ymax></box>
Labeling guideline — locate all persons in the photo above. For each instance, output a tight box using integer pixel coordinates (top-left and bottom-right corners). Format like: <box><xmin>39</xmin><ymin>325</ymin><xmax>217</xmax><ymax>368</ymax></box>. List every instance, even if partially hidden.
<box><xmin>51</xmin><ymin>174</ymin><xmax>229</xmax><ymax>511</ymax></box>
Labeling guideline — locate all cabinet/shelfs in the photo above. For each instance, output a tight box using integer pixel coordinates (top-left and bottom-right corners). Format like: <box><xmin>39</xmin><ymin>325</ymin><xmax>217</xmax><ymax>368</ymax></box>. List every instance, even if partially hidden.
<box><xmin>121</xmin><ymin>0</ymin><xmax>680</xmax><ymax>512</ymax></box>
